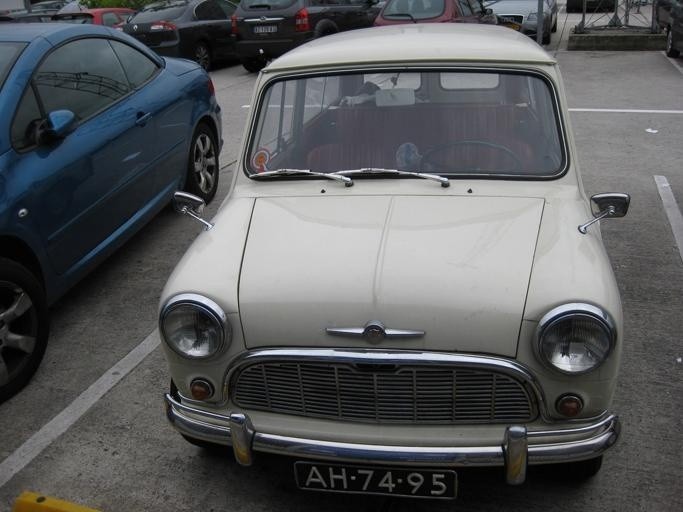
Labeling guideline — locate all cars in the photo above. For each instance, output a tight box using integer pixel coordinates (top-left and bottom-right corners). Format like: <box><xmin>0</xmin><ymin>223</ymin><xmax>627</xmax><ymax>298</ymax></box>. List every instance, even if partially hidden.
<box><xmin>482</xmin><ymin>0</ymin><xmax>558</xmax><ymax>45</ymax></box>
<box><xmin>370</xmin><ymin>0</ymin><xmax>501</xmax><ymax>26</ymax></box>
<box><xmin>156</xmin><ymin>20</ymin><xmax>631</xmax><ymax>503</ymax></box>
<box><xmin>0</xmin><ymin>19</ymin><xmax>225</xmax><ymax>409</ymax></box>
<box><xmin>0</xmin><ymin>0</ymin><xmax>138</xmax><ymax>31</ymax></box>
<box><xmin>652</xmin><ymin>0</ymin><xmax>683</xmax><ymax>57</ymax></box>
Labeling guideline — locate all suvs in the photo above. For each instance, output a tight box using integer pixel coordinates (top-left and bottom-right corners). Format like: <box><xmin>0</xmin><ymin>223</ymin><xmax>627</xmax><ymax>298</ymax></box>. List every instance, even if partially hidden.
<box><xmin>121</xmin><ymin>0</ymin><xmax>242</xmax><ymax>73</ymax></box>
<box><xmin>230</xmin><ymin>0</ymin><xmax>384</xmax><ymax>73</ymax></box>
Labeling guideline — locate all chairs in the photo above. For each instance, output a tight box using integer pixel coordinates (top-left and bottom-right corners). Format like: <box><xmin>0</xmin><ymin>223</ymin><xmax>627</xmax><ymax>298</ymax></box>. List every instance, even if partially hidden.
<box><xmin>304</xmin><ymin>104</ymin><xmax>535</xmax><ymax>174</ymax></box>
